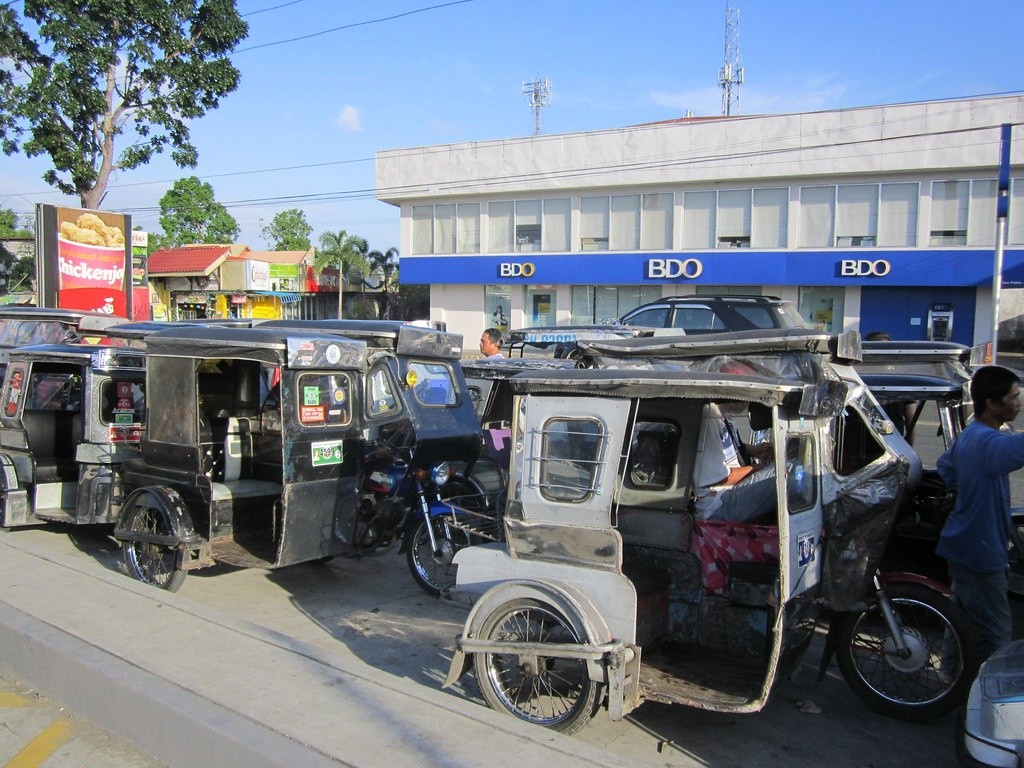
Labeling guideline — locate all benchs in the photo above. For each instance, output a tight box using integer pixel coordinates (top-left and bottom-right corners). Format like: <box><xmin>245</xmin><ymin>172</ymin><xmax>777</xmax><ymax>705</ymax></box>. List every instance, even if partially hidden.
<box><xmin>626</xmin><ymin>563</ymin><xmax>670</xmax><ymax>643</ymax></box>
<box><xmin>198</xmin><ymin>416</ymin><xmax>281</xmax><ymax>543</ymax></box>
<box><xmin>22</xmin><ymin>408</ymin><xmax>84</xmax><ymax>507</ymax></box>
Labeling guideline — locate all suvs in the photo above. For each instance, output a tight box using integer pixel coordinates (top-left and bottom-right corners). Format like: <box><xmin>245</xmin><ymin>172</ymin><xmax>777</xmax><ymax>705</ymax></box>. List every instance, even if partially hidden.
<box><xmin>611</xmin><ymin>294</ymin><xmax>813</xmax><ymax>337</ymax></box>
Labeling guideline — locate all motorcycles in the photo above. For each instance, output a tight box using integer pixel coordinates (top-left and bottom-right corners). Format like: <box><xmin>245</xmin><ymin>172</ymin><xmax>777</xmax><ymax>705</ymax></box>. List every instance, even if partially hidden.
<box><xmin>0</xmin><ymin>307</ymin><xmax>496</xmax><ymax>591</ymax></box>
<box><xmin>450</xmin><ymin>331</ymin><xmax>1024</xmax><ymax>768</ymax></box>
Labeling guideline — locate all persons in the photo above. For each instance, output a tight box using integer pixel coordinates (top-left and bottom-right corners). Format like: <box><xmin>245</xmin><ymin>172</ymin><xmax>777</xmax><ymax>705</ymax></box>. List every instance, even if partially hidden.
<box><xmin>478</xmin><ymin>327</ymin><xmax>505</xmax><ymax>359</ymax></box>
<box><xmin>692</xmin><ymin>361</ymin><xmax>814</xmax><ymax>523</ymax></box>
<box><xmin>932</xmin><ymin>365</ymin><xmax>1024</xmax><ymax>691</ymax></box>
<box><xmin>842</xmin><ymin>331</ymin><xmax>917</xmax><ymax>472</ymax></box>
